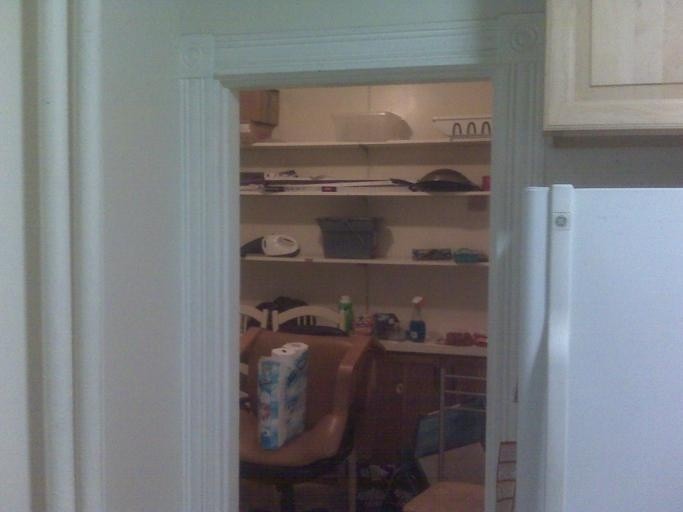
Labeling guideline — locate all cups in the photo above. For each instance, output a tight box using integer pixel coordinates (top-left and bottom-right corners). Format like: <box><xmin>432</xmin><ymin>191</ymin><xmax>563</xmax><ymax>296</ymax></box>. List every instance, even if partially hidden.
<box><xmin>261</xmin><ymin>232</ymin><xmax>300</xmax><ymax>257</ymax></box>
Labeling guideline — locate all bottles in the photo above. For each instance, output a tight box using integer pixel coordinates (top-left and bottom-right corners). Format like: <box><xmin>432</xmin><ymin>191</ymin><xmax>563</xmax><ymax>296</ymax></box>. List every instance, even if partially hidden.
<box><xmin>337</xmin><ymin>295</ymin><xmax>353</xmax><ymax>336</ymax></box>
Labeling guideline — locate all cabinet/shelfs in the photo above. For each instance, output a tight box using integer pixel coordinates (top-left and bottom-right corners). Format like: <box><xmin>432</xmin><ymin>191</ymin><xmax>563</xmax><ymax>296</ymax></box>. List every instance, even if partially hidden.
<box><xmin>240</xmin><ymin>139</ymin><xmax>492</xmax><ymax>356</ymax></box>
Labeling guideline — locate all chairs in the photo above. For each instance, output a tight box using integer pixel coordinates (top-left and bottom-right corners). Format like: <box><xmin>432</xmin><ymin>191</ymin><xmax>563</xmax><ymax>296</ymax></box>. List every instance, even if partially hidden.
<box><xmin>238</xmin><ymin>302</ymin><xmax>346</xmax><ymax>339</ymax></box>
<box><xmin>238</xmin><ymin>328</ymin><xmax>378</xmax><ymax>511</ymax></box>
<box><xmin>436</xmin><ymin>368</ymin><xmax>487</xmax><ymax>478</ymax></box>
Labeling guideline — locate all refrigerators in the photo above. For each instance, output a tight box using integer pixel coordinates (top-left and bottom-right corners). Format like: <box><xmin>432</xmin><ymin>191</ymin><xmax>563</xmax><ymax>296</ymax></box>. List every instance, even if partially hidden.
<box><xmin>515</xmin><ymin>178</ymin><xmax>680</xmax><ymax>512</ymax></box>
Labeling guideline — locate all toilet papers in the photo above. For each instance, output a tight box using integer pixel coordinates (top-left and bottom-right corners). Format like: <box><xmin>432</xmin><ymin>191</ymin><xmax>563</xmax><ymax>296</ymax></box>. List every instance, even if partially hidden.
<box><xmin>254</xmin><ymin>341</ymin><xmax>312</xmax><ymax>449</ymax></box>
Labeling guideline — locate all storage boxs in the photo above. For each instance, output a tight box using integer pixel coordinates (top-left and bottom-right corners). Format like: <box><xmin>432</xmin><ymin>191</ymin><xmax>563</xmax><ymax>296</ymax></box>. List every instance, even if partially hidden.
<box><xmin>240</xmin><ymin>89</ymin><xmax>280</xmax><ymax>142</ymax></box>
